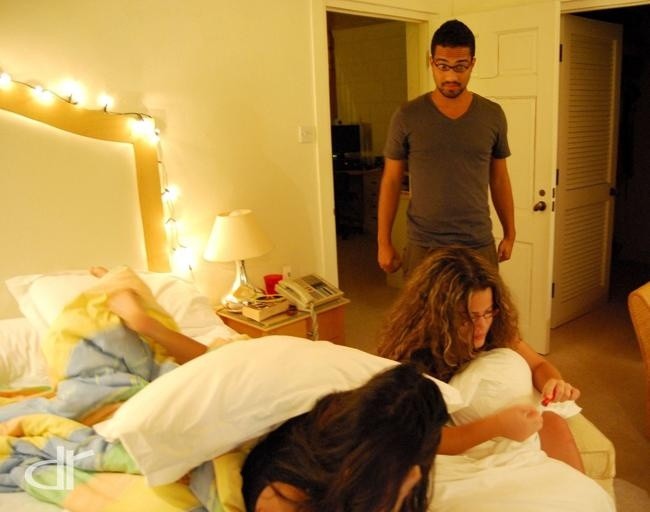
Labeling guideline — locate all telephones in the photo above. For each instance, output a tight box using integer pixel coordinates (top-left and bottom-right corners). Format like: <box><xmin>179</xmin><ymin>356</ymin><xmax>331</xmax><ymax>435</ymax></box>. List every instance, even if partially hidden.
<box><xmin>274</xmin><ymin>272</ymin><xmax>345</xmax><ymax>313</ymax></box>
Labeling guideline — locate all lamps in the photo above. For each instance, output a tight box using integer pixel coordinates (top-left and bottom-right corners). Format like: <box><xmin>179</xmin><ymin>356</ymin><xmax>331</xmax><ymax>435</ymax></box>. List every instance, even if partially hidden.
<box><xmin>200</xmin><ymin>203</ymin><xmax>271</xmax><ymax>307</ymax></box>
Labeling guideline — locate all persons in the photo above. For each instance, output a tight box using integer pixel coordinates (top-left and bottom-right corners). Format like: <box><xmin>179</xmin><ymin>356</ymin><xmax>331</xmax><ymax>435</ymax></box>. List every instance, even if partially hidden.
<box><xmin>377</xmin><ymin>245</ymin><xmax>585</xmax><ymax>474</ymax></box>
<box><xmin>376</xmin><ymin>19</ymin><xmax>516</xmax><ymax>282</ymax></box>
<box><xmin>107</xmin><ymin>290</ymin><xmax>451</xmax><ymax>512</ymax></box>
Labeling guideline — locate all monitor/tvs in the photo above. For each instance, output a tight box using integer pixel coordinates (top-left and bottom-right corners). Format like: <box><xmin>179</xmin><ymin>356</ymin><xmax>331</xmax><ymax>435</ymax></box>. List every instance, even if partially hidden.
<box><xmin>331</xmin><ymin>124</ymin><xmax>360</xmax><ymax>160</ymax></box>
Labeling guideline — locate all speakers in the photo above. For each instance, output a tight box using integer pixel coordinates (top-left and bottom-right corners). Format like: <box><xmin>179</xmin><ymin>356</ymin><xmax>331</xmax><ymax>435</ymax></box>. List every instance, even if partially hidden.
<box><xmin>375</xmin><ymin>156</ymin><xmax>384</xmax><ymax>169</ymax></box>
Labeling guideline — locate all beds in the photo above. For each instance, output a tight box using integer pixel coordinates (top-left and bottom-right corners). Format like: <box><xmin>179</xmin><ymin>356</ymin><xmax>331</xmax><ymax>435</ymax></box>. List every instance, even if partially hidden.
<box><xmin>1</xmin><ymin>73</ymin><xmax>615</xmax><ymax>511</ymax></box>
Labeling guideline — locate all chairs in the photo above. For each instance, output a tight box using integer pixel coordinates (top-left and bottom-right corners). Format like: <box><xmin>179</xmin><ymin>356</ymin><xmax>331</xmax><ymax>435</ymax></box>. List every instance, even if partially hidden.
<box><xmin>624</xmin><ymin>279</ymin><xmax>649</xmax><ymax>357</ymax></box>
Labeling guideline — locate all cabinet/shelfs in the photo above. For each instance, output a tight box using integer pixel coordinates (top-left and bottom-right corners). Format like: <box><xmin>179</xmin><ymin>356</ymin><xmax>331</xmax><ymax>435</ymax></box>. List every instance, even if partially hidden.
<box><xmin>362</xmin><ymin>174</ymin><xmax>380</xmax><ymax>235</ymax></box>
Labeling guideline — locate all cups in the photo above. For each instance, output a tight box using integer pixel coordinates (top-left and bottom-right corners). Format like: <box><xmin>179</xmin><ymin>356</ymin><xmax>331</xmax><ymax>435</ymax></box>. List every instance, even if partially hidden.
<box><xmin>263</xmin><ymin>274</ymin><xmax>283</xmax><ymax>295</ymax></box>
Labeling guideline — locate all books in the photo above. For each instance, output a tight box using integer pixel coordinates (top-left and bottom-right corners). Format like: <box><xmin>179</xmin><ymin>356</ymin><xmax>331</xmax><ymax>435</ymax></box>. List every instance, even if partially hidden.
<box><xmin>242</xmin><ymin>298</ymin><xmax>289</xmax><ymax>322</ymax></box>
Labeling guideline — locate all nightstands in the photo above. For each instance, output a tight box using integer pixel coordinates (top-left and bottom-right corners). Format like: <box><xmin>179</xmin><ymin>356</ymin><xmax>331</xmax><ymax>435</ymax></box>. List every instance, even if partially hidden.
<box><xmin>217</xmin><ymin>289</ymin><xmax>350</xmax><ymax>349</ymax></box>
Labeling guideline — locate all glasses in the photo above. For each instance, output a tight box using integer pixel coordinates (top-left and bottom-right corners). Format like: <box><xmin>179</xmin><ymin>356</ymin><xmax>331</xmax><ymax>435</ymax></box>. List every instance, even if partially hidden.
<box><xmin>433</xmin><ymin>58</ymin><xmax>473</xmax><ymax>72</ymax></box>
<box><xmin>465</xmin><ymin>309</ymin><xmax>500</xmax><ymax>324</ymax></box>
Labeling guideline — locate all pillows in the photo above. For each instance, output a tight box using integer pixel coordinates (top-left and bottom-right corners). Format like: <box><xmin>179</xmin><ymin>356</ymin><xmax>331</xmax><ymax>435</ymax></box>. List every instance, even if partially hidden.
<box><xmin>0</xmin><ymin>315</ymin><xmax>41</xmax><ymax>389</ymax></box>
<box><xmin>4</xmin><ymin>266</ymin><xmax>240</xmax><ymax>374</ymax></box>
